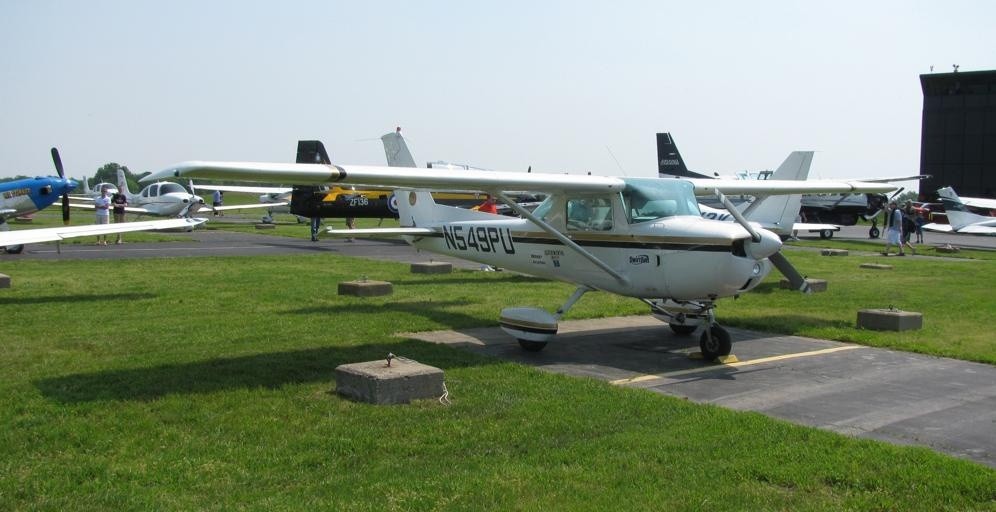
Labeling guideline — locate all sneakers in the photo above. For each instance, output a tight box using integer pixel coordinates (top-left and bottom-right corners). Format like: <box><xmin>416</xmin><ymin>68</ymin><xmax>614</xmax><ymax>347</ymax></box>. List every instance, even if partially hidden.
<box><xmin>95</xmin><ymin>238</ymin><xmax>123</xmax><ymax>246</ymax></box>
<box><xmin>911</xmin><ymin>245</ymin><xmax>917</xmax><ymax>255</ymax></box>
<box><xmin>880</xmin><ymin>252</ymin><xmax>905</xmax><ymax>257</ymax></box>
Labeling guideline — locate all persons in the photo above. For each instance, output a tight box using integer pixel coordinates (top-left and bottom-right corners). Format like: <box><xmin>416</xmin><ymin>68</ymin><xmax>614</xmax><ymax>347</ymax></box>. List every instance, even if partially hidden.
<box><xmin>899</xmin><ymin>208</ymin><xmax>917</xmax><ymax>257</ymax></box>
<box><xmin>882</xmin><ymin>198</ymin><xmax>930</xmax><ymax>245</ymax></box>
<box><xmin>94</xmin><ymin>187</ymin><xmax>111</xmax><ymax>246</ymax></box>
<box><xmin>212</xmin><ymin>190</ymin><xmax>224</xmax><ymax>217</ymax></box>
<box><xmin>109</xmin><ymin>186</ymin><xmax>127</xmax><ymax>245</ymax></box>
<box><xmin>879</xmin><ymin>201</ymin><xmax>905</xmax><ymax>258</ymax></box>
<box><xmin>477</xmin><ymin>194</ymin><xmax>504</xmax><ymax>273</ymax></box>
<box><xmin>344</xmin><ymin>218</ymin><xmax>355</xmax><ymax>242</ymax></box>
<box><xmin>571</xmin><ymin>199</ymin><xmax>594</xmax><ymax>232</ymax></box>
